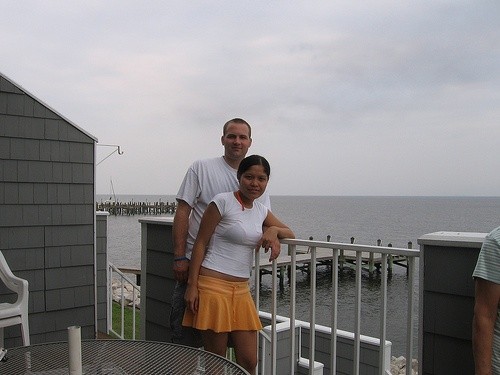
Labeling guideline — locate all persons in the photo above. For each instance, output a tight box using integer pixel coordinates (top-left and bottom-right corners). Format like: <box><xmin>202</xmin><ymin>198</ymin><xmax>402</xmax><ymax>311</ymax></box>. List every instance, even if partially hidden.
<box><xmin>171</xmin><ymin>118</ymin><xmax>281</xmax><ymax>375</ymax></box>
<box><xmin>183</xmin><ymin>155</ymin><xmax>295</xmax><ymax>375</ymax></box>
<box><xmin>471</xmin><ymin>227</ymin><xmax>500</xmax><ymax>375</ymax></box>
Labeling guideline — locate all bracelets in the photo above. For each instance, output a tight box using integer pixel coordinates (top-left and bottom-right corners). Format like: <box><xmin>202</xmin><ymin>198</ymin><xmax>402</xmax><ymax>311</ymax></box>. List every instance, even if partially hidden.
<box><xmin>173</xmin><ymin>256</ymin><xmax>186</xmax><ymax>261</ymax></box>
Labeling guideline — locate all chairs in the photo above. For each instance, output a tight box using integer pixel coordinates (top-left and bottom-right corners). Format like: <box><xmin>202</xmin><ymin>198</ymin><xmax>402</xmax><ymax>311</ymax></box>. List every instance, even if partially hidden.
<box><xmin>0</xmin><ymin>250</ymin><xmax>31</xmax><ymax>370</ymax></box>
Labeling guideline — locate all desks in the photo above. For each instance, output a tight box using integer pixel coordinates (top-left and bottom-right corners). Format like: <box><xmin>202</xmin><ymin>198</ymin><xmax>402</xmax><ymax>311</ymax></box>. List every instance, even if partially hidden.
<box><xmin>0</xmin><ymin>339</ymin><xmax>249</xmax><ymax>375</ymax></box>
